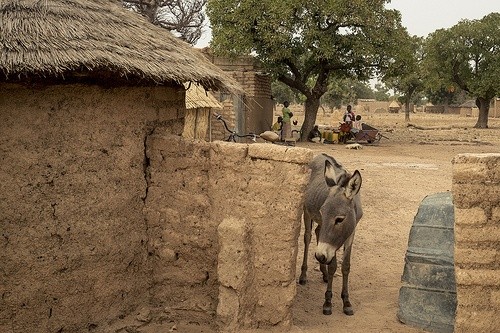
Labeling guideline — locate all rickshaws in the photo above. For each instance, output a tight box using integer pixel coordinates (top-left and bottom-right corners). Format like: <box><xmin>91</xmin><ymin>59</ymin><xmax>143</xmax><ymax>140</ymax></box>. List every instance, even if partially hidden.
<box><xmin>350</xmin><ymin>123</ymin><xmax>390</xmax><ymax>144</ymax></box>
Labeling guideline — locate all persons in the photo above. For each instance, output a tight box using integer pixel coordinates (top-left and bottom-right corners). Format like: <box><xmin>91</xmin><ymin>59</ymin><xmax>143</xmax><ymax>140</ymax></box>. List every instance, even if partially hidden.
<box><xmin>343</xmin><ymin>104</ymin><xmax>356</xmax><ymax>138</ymax></box>
<box><xmin>272</xmin><ymin>115</ymin><xmax>284</xmax><ymax>142</ymax></box>
<box><xmin>350</xmin><ymin>114</ymin><xmax>362</xmax><ymax>138</ymax></box>
<box><xmin>309</xmin><ymin>125</ymin><xmax>322</xmax><ymax>143</ymax></box>
<box><xmin>281</xmin><ymin>100</ymin><xmax>294</xmax><ymax>141</ymax></box>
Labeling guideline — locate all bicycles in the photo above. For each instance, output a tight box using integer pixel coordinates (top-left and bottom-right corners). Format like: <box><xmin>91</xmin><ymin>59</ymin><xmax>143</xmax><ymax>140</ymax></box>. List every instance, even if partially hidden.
<box><xmin>213</xmin><ymin>113</ymin><xmax>297</xmax><ymax>146</ymax></box>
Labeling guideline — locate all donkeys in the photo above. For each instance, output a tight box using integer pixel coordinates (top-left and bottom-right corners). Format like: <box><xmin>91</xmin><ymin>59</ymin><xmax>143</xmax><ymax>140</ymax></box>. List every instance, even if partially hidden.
<box><xmin>299</xmin><ymin>152</ymin><xmax>363</xmax><ymax>315</ymax></box>
